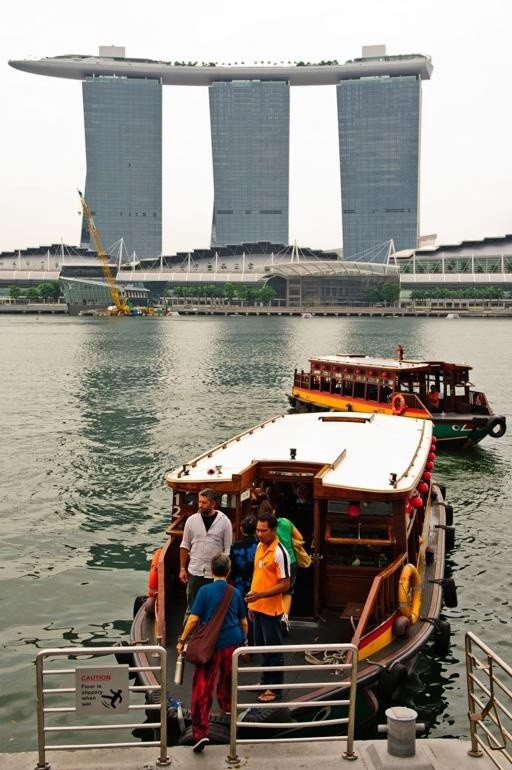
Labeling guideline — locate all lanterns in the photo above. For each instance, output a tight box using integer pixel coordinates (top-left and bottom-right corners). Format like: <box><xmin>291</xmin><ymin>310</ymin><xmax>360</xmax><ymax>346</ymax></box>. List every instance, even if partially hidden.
<box><xmin>347</xmin><ymin>505</ymin><xmax>361</xmax><ymax>518</ymax></box>
<box><xmin>311</xmin><ymin>363</ymin><xmax>389</xmax><ymax>377</ymax></box>
<box><xmin>410</xmin><ymin>436</ymin><xmax>437</xmax><ymax>508</ymax></box>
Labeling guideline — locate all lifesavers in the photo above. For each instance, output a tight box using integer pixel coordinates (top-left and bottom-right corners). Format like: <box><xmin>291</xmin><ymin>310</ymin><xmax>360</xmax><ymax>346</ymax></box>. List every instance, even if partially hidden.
<box><xmin>392</xmin><ymin>394</ymin><xmax>406</xmax><ymax>414</ymax></box>
<box><xmin>149</xmin><ymin>549</ymin><xmax>162</xmax><ymax>598</ymax></box>
<box><xmin>398</xmin><ymin>563</ymin><xmax>422</xmax><ymax>624</ymax></box>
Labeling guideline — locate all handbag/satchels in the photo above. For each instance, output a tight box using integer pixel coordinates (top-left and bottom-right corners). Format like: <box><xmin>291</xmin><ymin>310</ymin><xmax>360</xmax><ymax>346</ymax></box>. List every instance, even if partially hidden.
<box><xmin>292</xmin><ymin>539</ymin><xmax>312</xmax><ymax>570</ymax></box>
<box><xmin>185</xmin><ymin>624</ymin><xmax>218</xmax><ymax>664</ymax></box>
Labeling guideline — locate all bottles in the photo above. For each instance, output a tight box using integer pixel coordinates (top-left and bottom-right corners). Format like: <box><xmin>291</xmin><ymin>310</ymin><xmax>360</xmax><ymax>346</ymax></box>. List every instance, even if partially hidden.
<box><xmin>172</xmin><ymin>656</ymin><xmax>184</xmax><ymax>685</ymax></box>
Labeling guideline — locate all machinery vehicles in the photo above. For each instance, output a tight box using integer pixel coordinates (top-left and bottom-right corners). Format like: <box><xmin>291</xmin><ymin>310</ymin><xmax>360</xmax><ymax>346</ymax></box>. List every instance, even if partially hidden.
<box><xmin>77</xmin><ymin>190</ymin><xmax>155</xmax><ymax>317</ymax></box>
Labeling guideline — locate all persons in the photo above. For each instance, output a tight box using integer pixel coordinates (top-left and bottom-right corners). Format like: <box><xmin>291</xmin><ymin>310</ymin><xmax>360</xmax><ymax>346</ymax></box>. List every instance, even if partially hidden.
<box><xmin>292</xmin><ymin>482</ymin><xmax>313</xmax><ymax>504</ymax></box>
<box><xmin>230</xmin><ymin>515</ymin><xmax>260</xmax><ymax>663</ymax></box>
<box><xmin>179</xmin><ymin>488</ymin><xmax>233</xmax><ymax>635</ymax></box>
<box><xmin>243</xmin><ymin>512</ymin><xmax>292</xmax><ymax>703</ymax></box>
<box><xmin>426</xmin><ymin>385</ymin><xmax>439</xmax><ymax>410</ymax></box>
<box><xmin>257</xmin><ymin>500</ymin><xmax>304</xmax><ymax>636</ymax></box>
<box><xmin>176</xmin><ymin>554</ymin><xmax>248</xmax><ymax>752</ymax></box>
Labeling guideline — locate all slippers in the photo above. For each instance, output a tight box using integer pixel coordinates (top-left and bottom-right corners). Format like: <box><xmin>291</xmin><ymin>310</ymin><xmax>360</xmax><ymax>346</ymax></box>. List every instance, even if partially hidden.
<box><xmin>258</xmin><ymin>691</ymin><xmax>280</xmax><ymax>702</ymax></box>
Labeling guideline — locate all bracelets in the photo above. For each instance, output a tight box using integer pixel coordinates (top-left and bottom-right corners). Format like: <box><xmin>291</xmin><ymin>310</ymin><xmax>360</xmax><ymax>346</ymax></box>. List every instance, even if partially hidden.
<box><xmin>178</xmin><ymin>638</ymin><xmax>185</xmax><ymax>644</ymax></box>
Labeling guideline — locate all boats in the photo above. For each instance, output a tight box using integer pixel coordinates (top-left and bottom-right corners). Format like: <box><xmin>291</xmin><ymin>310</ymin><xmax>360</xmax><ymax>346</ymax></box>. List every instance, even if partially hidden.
<box><xmin>283</xmin><ymin>346</ymin><xmax>506</xmax><ymax>451</ymax></box>
<box><xmin>303</xmin><ymin>313</ymin><xmax>312</xmax><ymax>319</ymax></box>
<box><xmin>445</xmin><ymin>313</ymin><xmax>459</xmax><ymax>319</ymax></box>
<box><xmin>129</xmin><ymin>405</ymin><xmax>457</xmax><ymax>744</ymax></box>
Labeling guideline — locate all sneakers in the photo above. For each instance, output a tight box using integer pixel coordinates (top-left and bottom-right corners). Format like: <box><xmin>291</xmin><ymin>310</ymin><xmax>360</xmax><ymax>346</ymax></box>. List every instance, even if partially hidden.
<box><xmin>281</xmin><ymin>614</ymin><xmax>289</xmax><ymax>634</ymax></box>
<box><xmin>192</xmin><ymin>737</ymin><xmax>209</xmax><ymax>751</ymax></box>
